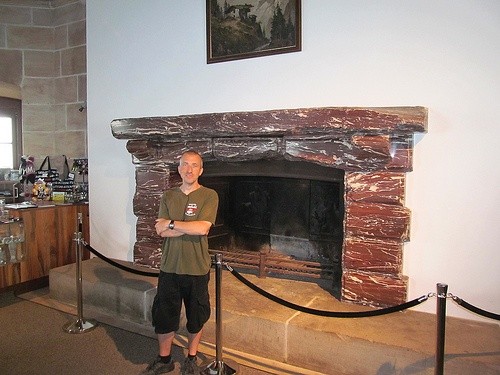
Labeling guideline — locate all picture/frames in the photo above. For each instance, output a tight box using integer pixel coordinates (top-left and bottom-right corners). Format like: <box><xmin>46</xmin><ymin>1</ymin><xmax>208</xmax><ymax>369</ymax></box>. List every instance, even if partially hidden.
<box><xmin>206</xmin><ymin>0</ymin><xmax>302</xmax><ymax>64</ymax></box>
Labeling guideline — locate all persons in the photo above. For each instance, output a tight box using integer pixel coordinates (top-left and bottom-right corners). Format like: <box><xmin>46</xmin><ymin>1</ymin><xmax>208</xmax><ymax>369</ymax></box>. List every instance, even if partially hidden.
<box><xmin>143</xmin><ymin>150</ymin><xmax>219</xmax><ymax>375</ymax></box>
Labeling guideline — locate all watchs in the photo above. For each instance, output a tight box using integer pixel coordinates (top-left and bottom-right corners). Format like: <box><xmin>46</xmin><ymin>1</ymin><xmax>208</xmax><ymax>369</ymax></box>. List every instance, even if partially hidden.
<box><xmin>169</xmin><ymin>220</ymin><xmax>175</xmax><ymax>230</ymax></box>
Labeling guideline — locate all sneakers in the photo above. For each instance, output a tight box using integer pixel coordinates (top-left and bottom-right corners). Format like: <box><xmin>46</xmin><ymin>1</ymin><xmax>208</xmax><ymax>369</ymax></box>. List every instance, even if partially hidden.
<box><xmin>144</xmin><ymin>356</ymin><xmax>174</xmax><ymax>374</ymax></box>
<box><xmin>184</xmin><ymin>356</ymin><xmax>202</xmax><ymax>375</ymax></box>
